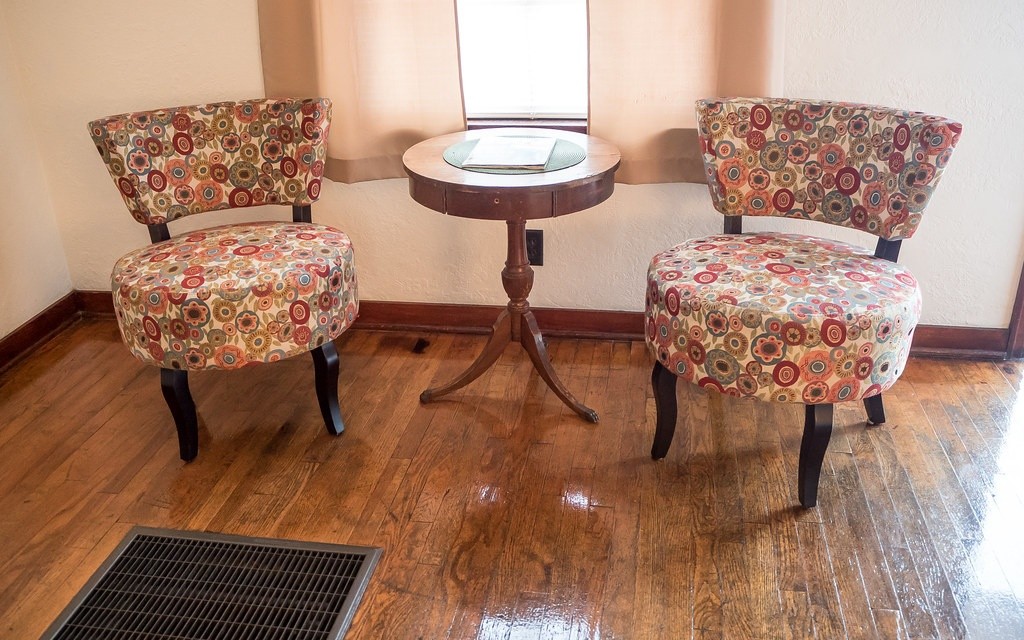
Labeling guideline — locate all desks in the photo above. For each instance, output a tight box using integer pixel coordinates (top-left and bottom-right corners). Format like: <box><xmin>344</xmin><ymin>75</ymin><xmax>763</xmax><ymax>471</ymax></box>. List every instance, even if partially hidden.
<box><xmin>401</xmin><ymin>129</ymin><xmax>624</xmax><ymax>425</ymax></box>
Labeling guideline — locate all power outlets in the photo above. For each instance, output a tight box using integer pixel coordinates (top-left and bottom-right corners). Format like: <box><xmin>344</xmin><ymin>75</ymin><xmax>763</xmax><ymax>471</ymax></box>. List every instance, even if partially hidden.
<box><xmin>525</xmin><ymin>230</ymin><xmax>544</xmax><ymax>266</ymax></box>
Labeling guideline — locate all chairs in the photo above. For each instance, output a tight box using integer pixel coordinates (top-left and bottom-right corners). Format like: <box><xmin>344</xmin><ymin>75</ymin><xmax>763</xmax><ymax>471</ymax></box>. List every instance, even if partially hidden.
<box><xmin>644</xmin><ymin>96</ymin><xmax>962</xmax><ymax>510</ymax></box>
<box><xmin>88</xmin><ymin>98</ymin><xmax>353</xmax><ymax>464</ymax></box>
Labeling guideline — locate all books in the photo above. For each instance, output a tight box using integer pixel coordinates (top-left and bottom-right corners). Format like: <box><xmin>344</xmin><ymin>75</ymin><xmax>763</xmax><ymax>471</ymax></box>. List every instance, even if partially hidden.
<box><xmin>462</xmin><ymin>133</ymin><xmax>558</xmax><ymax>169</ymax></box>
